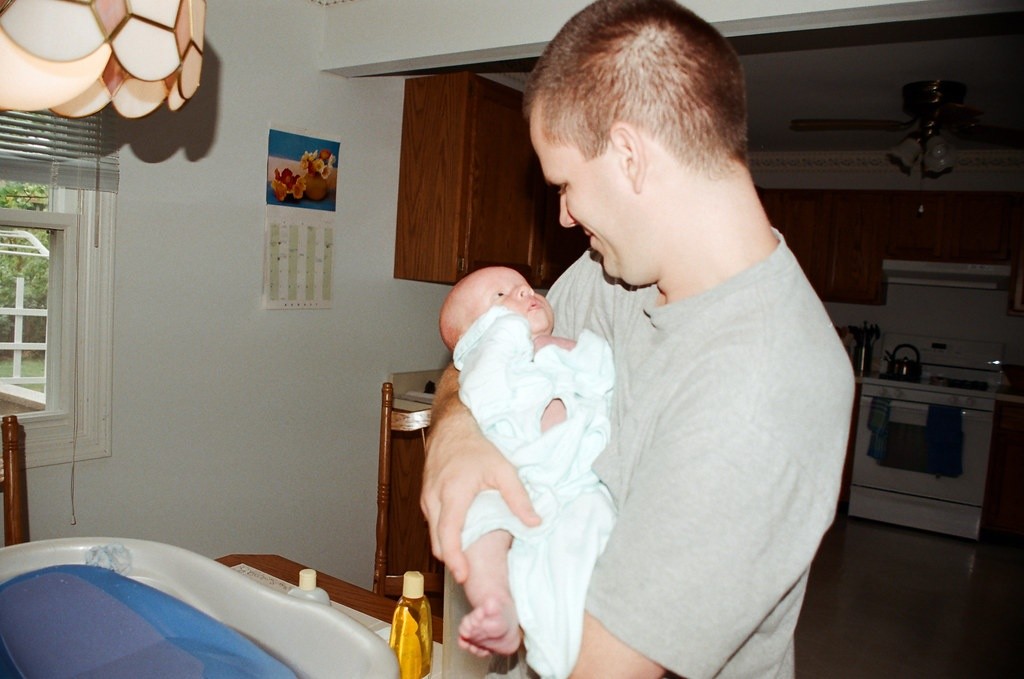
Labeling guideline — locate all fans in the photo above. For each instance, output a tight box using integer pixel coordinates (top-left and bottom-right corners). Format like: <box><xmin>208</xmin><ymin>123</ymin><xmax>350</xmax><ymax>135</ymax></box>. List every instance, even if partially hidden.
<box><xmin>792</xmin><ymin>81</ymin><xmax>1024</xmax><ymax>149</ymax></box>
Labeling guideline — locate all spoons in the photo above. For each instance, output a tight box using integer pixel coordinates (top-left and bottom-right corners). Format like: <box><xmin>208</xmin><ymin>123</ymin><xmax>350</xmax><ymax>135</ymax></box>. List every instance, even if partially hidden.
<box><xmin>848</xmin><ymin>320</ymin><xmax>881</xmax><ymax>346</ymax></box>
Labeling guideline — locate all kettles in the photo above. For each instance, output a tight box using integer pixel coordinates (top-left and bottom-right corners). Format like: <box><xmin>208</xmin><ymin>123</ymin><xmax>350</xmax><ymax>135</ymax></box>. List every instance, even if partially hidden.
<box><xmin>882</xmin><ymin>343</ymin><xmax>922</xmax><ymax>381</ymax></box>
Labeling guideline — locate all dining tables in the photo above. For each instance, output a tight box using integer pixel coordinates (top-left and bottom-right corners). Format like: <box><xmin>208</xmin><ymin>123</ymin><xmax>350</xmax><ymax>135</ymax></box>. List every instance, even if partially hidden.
<box><xmin>215</xmin><ymin>554</ymin><xmax>443</xmax><ymax>679</ymax></box>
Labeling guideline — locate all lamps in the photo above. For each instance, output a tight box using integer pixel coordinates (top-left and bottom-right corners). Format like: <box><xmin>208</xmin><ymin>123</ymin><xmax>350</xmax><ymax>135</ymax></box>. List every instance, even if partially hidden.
<box><xmin>887</xmin><ymin>106</ymin><xmax>958</xmax><ymax>173</ymax></box>
<box><xmin>0</xmin><ymin>0</ymin><xmax>207</xmax><ymax>119</ymax></box>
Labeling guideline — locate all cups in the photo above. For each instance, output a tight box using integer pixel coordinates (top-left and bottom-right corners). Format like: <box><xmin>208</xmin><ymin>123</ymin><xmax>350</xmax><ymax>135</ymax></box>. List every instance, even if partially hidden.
<box><xmin>855</xmin><ymin>346</ymin><xmax>873</xmax><ymax>372</ymax></box>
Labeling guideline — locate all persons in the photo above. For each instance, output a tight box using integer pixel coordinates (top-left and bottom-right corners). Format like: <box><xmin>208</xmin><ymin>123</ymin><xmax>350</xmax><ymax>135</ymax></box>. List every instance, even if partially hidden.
<box><xmin>415</xmin><ymin>1</ymin><xmax>854</xmax><ymax>679</ymax></box>
<box><xmin>438</xmin><ymin>265</ymin><xmax>618</xmax><ymax>679</ymax></box>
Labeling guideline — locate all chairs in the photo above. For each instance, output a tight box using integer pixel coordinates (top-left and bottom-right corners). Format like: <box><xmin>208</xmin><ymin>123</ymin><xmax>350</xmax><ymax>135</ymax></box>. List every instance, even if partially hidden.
<box><xmin>373</xmin><ymin>385</ymin><xmax>445</xmax><ymax>618</ymax></box>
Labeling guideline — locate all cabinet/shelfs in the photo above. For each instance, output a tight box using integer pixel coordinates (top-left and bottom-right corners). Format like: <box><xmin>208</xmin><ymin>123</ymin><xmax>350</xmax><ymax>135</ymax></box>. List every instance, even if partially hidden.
<box><xmin>756</xmin><ymin>185</ymin><xmax>1023</xmax><ymax>316</ymax></box>
<box><xmin>394</xmin><ymin>72</ymin><xmax>590</xmax><ymax>290</ymax></box>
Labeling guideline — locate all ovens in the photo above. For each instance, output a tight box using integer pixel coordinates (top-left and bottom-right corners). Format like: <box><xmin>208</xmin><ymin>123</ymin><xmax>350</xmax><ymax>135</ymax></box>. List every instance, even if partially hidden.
<box><xmin>847</xmin><ymin>383</ymin><xmax>996</xmax><ymax>541</ymax></box>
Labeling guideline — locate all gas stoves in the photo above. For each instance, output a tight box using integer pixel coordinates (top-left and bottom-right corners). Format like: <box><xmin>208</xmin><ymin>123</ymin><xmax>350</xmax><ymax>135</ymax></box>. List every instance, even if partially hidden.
<box><xmin>855</xmin><ymin>331</ymin><xmax>1004</xmax><ymax>398</ymax></box>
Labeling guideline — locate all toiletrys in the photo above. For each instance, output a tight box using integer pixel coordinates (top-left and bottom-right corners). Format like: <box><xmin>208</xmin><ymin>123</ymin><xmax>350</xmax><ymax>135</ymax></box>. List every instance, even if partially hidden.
<box><xmin>279</xmin><ymin>569</ymin><xmax>434</xmax><ymax>679</ymax></box>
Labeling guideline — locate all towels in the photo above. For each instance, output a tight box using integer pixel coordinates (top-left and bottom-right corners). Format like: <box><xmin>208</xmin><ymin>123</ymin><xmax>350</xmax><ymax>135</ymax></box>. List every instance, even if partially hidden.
<box><xmin>865</xmin><ymin>396</ymin><xmax>965</xmax><ymax>479</ymax></box>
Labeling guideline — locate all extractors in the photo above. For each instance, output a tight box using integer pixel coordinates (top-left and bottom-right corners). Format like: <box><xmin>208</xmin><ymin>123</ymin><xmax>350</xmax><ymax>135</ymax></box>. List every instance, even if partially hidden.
<box><xmin>879</xmin><ymin>259</ymin><xmax>1016</xmax><ymax>290</ymax></box>
